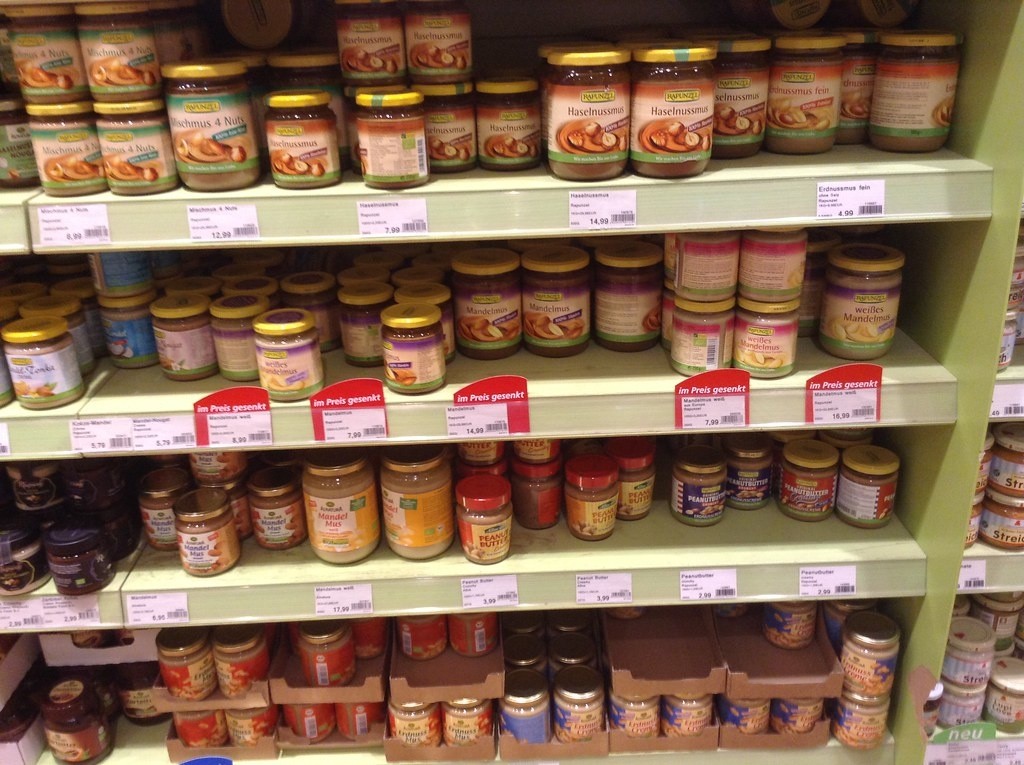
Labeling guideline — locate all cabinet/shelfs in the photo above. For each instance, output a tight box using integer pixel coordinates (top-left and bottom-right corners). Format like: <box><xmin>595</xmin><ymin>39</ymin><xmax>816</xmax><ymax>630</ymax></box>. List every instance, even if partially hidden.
<box><xmin>0</xmin><ymin>1</ymin><xmax>1024</xmax><ymax>765</ymax></box>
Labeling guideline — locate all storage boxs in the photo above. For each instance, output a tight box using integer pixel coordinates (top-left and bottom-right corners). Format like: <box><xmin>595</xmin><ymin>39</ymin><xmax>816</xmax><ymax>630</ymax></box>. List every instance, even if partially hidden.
<box><xmin>383</xmin><ymin>709</ymin><xmax>497</xmax><ymax>763</ymax></box>
<box><xmin>0</xmin><ymin>712</ymin><xmax>48</xmax><ymax>765</ymax></box>
<box><xmin>711</xmin><ymin>604</ymin><xmax>844</xmax><ymax>699</ymax></box>
<box><xmin>269</xmin><ymin>621</ymin><xmax>390</xmax><ymax>705</ymax></box>
<box><xmin>389</xmin><ymin>617</ymin><xmax>506</xmax><ymax>704</ymax></box>
<box><xmin>599</xmin><ymin>605</ymin><xmax>728</xmax><ymax>696</ymax></box>
<box><xmin>496</xmin><ymin>610</ymin><xmax>610</xmax><ymax>761</ymax></box>
<box><xmin>606</xmin><ymin>702</ymin><xmax>720</xmax><ymax>754</ymax></box>
<box><xmin>165</xmin><ymin>719</ymin><xmax>281</xmax><ymax>763</ymax></box>
<box><xmin>151</xmin><ymin>624</ymin><xmax>280</xmax><ymax>714</ymax></box>
<box><xmin>715</xmin><ymin>701</ymin><xmax>832</xmax><ymax>749</ymax></box>
<box><xmin>0</xmin><ymin>633</ymin><xmax>39</xmax><ymax>711</ymax></box>
<box><xmin>38</xmin><ymin>627</ymin><xmax>162</xmax><ymax>667</ymax></box>
<box><xmin>277</xmin><ymin>711</ymin><xmax>386</xmax><ymax>749</ymax></box>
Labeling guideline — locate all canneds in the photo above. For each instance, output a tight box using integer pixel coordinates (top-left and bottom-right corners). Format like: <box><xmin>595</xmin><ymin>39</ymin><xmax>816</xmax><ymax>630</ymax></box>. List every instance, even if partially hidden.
<box><xmin>0</xmin><ymin>227</ymin><xmax>906</xmax><ymax>410</ymax></box>
<box><xmin>0</xmin><ymin>427</ymin><xmax>900</xmax><ymax>765</ymax></box>
<box><xmin>922</xmin><ymin>216</ymin><xmax>1024</xmax><ymax>741</ymax></box>
<box><xmin>0</xmin><ymin>0</ymin><xmax>958</xmax><ymax>192</ymax></box>
